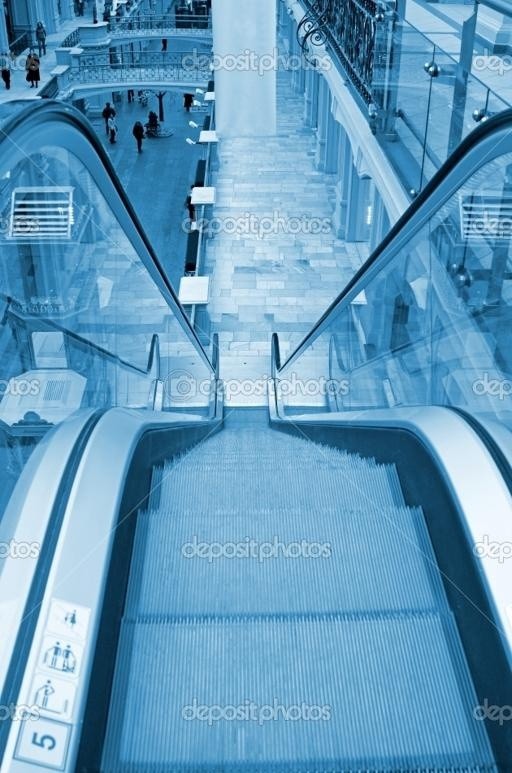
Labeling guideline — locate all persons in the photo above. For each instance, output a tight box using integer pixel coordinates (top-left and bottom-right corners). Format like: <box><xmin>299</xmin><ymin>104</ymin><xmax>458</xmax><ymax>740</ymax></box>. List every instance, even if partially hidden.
<box><xmin>0</xmin><ymin>0</ymin><xmax>195</xmax><ymax>154</ymax></box>
